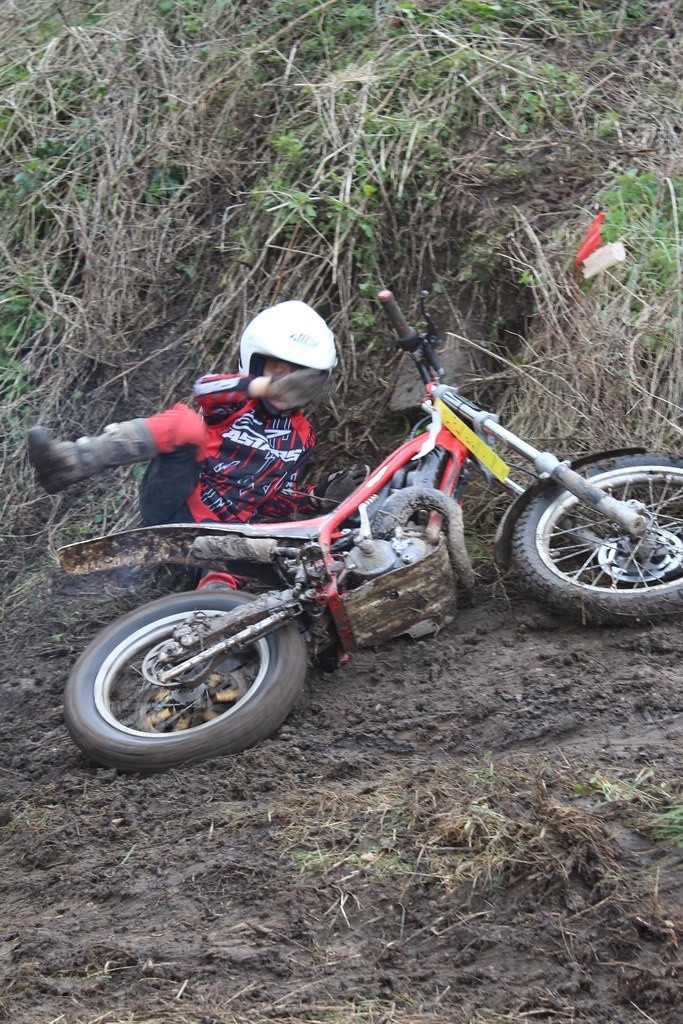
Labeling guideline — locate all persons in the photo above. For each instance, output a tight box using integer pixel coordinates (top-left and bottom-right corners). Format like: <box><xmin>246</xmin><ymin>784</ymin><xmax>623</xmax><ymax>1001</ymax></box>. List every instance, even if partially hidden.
<box><xmin>26</xmin><ymin>298</ymin><xmax>372</xmax><ymax>593</ymax></box>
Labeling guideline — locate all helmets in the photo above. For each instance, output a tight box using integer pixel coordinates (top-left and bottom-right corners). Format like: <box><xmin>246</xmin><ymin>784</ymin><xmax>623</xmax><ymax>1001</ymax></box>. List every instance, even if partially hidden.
<box><xmin>237</xmin><ymin>299</ymin><xmax>339</xmax><ymax>383</ymax></box>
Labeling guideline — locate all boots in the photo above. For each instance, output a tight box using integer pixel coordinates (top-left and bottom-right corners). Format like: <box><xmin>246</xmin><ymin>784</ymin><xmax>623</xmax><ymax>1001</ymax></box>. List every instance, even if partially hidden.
<box><xmin>27</xmin><ymin>416</ymin><xmax>158</xmax><ymax>496</ymax></box>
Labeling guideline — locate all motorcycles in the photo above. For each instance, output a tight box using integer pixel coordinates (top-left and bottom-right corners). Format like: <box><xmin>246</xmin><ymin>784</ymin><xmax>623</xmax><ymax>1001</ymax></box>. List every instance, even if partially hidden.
<box><xmin>57</xmin><ymin>291</ymin><xmax>683</xmax><ymax>774</ymax></box>
